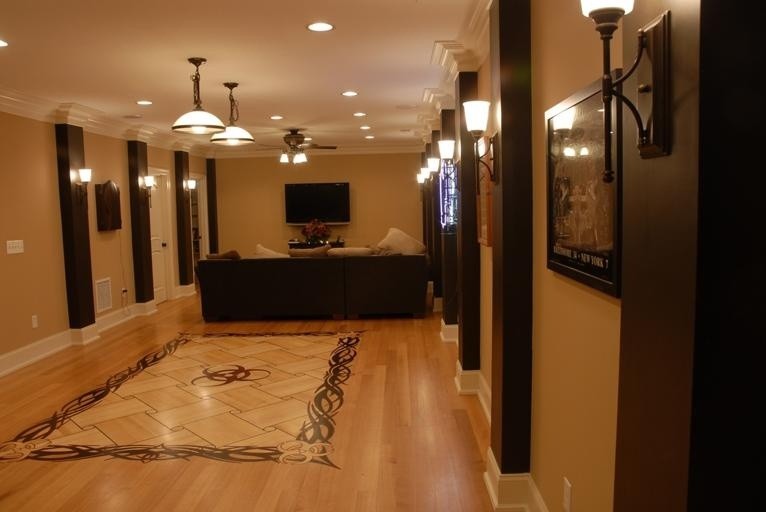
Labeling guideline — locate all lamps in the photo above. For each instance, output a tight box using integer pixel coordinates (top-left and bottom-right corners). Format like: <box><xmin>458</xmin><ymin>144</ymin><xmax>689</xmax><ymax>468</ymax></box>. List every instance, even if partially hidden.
<box><xmin>171</xmin><ymin>57</ymin><xmax>257</xmax><ymax>148</ymax></box>
<box><xmin>143</xmin><ymin>175</ymin><xmax>153</xmax><ymax>208</ymax></box>
<box><xmin>436</xmin><ymin>101</ymin><xmax>497</xmax><ymax>183</ymax></box>
<box><xmin>578</xmin><ymin>0</ymin><xmax>673</xmax><ymax>182</ymax></box>
<box><xmin>75</xmin><ymin>168</ymin><xmax>91</xmax><ymax>205</ymax></box>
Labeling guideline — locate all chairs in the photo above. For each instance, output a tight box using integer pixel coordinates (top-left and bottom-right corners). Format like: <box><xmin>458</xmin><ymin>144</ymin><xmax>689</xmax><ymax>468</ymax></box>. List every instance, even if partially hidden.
<box><xmin>345</xmin><ymin>255</ymin><xmax>434</xmax><ymax>320</ymax></box>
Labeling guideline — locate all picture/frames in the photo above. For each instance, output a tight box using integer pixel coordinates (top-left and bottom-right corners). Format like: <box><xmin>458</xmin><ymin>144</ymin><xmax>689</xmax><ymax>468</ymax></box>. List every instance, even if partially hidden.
<box><xmin>543</xmin><ymin>68</ymin><xmax>621</xmax><ymax>300</ymax></box>
<box><xmin>473</xmin><ymin>137</ymin><xmax>494</xmax><ymax>247</ymax></box>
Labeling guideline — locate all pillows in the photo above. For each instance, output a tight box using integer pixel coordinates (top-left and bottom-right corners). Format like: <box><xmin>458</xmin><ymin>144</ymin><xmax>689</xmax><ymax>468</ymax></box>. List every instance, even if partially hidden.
<box><xmin>205</xmin><ymin>227</ymin><xmax>427</xmax><ymax>259</ymax></box>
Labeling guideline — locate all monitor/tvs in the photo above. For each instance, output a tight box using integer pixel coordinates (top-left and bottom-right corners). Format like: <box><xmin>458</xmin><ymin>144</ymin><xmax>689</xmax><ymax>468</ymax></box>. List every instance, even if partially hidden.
<box><xmin>284</xmin><ymin>183</ymin><xmax>350</xmax><ymax>226</ymax></box>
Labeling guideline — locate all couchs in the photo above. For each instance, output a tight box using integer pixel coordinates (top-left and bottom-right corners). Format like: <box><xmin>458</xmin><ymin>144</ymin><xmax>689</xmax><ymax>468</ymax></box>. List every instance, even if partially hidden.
<box><xmin>196</xmin><ymin>255</ymin><xmax>345</xmax><ymax>322</ymax></box>
